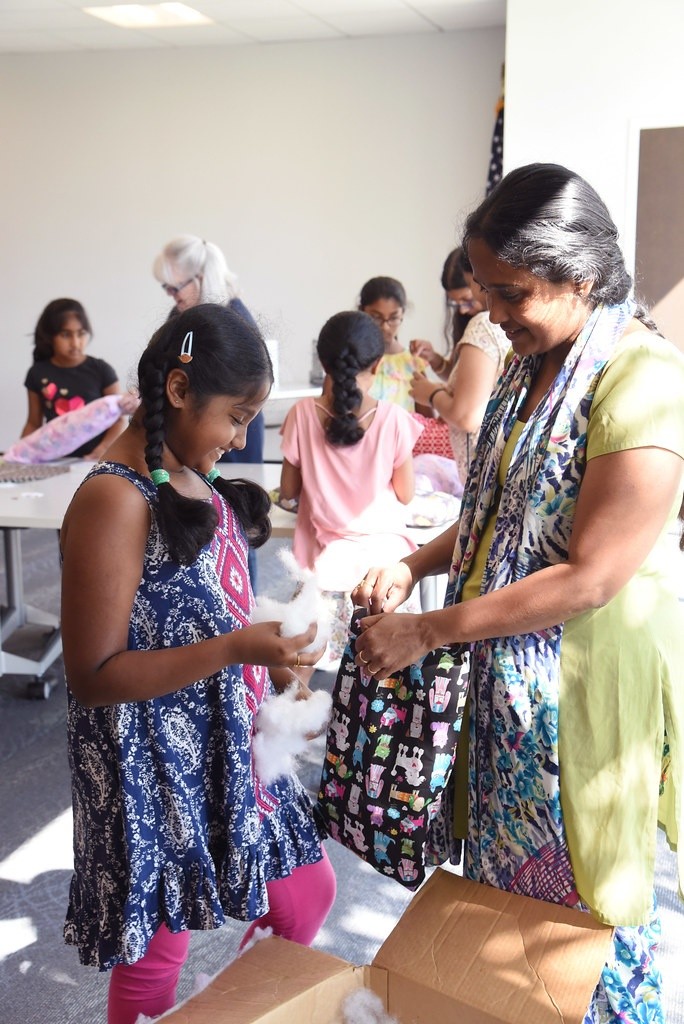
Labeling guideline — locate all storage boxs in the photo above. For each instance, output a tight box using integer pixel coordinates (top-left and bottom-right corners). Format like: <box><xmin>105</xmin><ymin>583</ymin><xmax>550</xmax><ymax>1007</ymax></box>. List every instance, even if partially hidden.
<box><xmin>143</xmin><ymin>864</ymin><xmax>617</xmax><ymax>1024</ymax></box>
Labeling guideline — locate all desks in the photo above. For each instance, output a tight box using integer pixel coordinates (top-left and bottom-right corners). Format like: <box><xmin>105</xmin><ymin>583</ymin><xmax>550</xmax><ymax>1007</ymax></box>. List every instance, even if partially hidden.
<box><xmin>263</xmin><ymin>386</ymin><xmax>325</xmax><ymax>427</ymax></box>
<box><xmin>0</xmin><ymin>462</ymin><xmax>459</xmax><ymax>701</ymax></box>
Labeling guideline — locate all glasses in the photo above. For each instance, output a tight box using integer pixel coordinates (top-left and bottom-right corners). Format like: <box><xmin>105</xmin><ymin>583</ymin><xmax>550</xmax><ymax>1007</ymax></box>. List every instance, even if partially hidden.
<box><xmin>361</xmin><ymin>309</ymin><xmax>404</xmax><ymax>328</ymax></box>
<box><xmin>445</xmin><ymin>291</ymin><xmax>475</xmax><ymax>309</ymax></box>
<box><xmin>162</xmin><ymin>273</ymin><xmax>199</xmax><ymax>297</ymax></box>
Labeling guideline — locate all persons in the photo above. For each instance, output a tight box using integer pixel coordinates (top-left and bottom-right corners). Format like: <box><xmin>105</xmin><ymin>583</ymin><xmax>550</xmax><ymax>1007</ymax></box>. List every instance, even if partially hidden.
<box><xmin>407</xmin><ymin>246</ymin><xmax>515</xmax><ymax>493</ymax></box>
<box><xmin>359</xmin><ymin>277</ymin><xmax>435</xmax><ymax>420</ymax></box>
<box><xmin>118</xmin><ymin>235</ymin><xmax>266</xmax><ymax>600</ymax></box>
<box><xmin>20</xmin><ymin>298</ymin><xmax>131</xmax><ymax>463</ymax></box>
<box><xmin>279</xmin><ymin>310</ymin><xmax>424</xmax><ymax>571</ymax></box>
<box><xmin>350</xmin><ymin>163</ymin><xmax>684</xmax><ymax>1024</ymax></box>
<box><xmin>55</xmin><ymin>304</ymin><xmax>336</xmax><ymax>1024</ymax></box>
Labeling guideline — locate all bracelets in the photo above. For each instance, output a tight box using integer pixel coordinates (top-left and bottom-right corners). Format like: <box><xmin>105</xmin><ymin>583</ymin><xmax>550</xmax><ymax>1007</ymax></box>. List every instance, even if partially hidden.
<box><xmin>438</xmin><ymin>361</ymin><xmax>447</xmax><ymax>374</ymax></box>
<box><xmin>429</xmin><ymin>387</ymin><xmax>445</xmax><ymax>404</ymax></box>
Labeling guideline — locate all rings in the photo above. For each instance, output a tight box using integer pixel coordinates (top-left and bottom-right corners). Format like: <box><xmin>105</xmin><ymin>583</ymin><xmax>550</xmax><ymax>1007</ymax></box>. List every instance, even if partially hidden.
<box><xmin>367</xmin><ymin>665</ymin><xmax>377</xmax><ymax>674</ymax></box>
<box><xmin>297</xmin><ymin>656</ymin><xmax>300</xmax><ymax>666</ymax></box>
<box><xmin>357</xmin><ymin>580</ymin><xmax>364</xmax><ymax>588</ymax></box>
<box><xmin>359</xmin><ymin>651</ymin><xmax>368</xmax><ymax>664</ymax></box>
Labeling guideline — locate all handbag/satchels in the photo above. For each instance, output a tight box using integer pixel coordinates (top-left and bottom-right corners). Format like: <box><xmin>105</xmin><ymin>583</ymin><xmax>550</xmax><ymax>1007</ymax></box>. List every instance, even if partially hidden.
<box><xmin>317</xmin><ymin>607</ymin><xmax>471</xmax><ymax>891</ymax></box>
